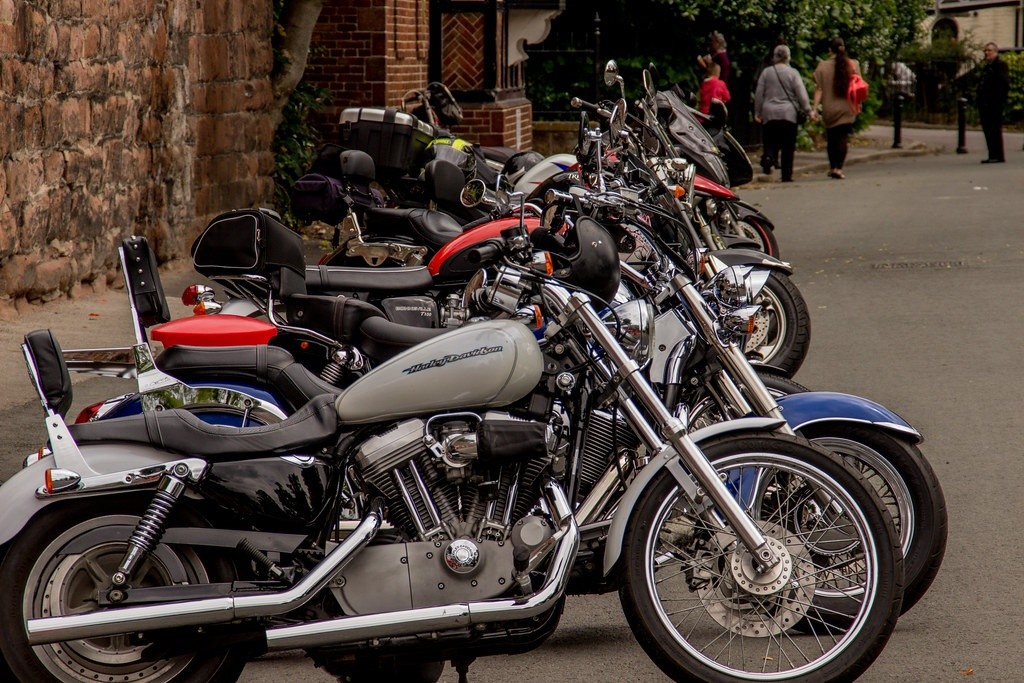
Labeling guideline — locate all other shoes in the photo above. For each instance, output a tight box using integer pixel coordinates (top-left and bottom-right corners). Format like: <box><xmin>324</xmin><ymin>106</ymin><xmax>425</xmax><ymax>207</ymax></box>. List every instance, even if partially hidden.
<box><xmin>982</xmin><ymin>159</ymin><xmax>1004</xmax><ymax>163</ymax></box>
<box><xmin>782</xmin><ymin>179</ymin><xmax>792</xmax><ymax>182</ymax></box>
<box><xmin>828</xmin><ymin>168</ymin><xmax>845</xmax><ymax>179</ymax></box>
<box><xmin>763</xmin><ymin>157</ymin><xmax>770</xmax><ymax>174</ymax></box>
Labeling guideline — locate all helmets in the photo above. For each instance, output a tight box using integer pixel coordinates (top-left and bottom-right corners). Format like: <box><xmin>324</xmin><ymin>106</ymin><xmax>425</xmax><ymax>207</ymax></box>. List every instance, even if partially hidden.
<box><xmin>550</xmin><ymin>215</ymin><xmax>621</xmax><ymax>313</ymax></box>
<box><xmin>501</xmin><ymin>151</ymin><xmax>545</xmax><ymax>193</ymax></box>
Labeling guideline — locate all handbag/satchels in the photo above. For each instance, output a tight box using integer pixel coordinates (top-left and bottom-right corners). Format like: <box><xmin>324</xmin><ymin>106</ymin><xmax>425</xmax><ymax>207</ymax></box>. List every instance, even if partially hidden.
<box><xmin>191</xmin><ymin>207</ymin><xmax>306</xmax><ymax>298</ymax></box>
<box><xmin>796</xmin><ymin>111</ymin><xmax>806</xmax><ymax>124</ymax></box>
<box><xmin>847</xmin><ymin>60</ymin><xmax>869</xmax><ymax>113</ymax></box>
<box><xmin>290</xmin><ymin>144</ymin><xmax>385</xmax><ymax>227</ymax></box>
<box><xmin>423</xmin><ymin>135</ymin><xmax>498</xmax><ymax>184</ymax></box>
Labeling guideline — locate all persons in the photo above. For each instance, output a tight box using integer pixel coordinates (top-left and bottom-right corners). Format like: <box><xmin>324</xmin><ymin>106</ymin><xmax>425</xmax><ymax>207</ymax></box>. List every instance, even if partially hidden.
<box><xmin>976</xmin><ymin>42</ymin><xmax>1010</xmax><ymax>163</ymax></box>
<box><xmin>754</xmin><ymin>45</ymin><xmax>819</xmax><ymax>183</ymax></box>
<box><xmin>812</xmin><ymin>38</ymin><xmax>863</xmax><ymax>178</ymax></box>
<box><xmin>694</xmin><ymin>30</ymin><xmax>731</xmax><ymax>124</ymax></box>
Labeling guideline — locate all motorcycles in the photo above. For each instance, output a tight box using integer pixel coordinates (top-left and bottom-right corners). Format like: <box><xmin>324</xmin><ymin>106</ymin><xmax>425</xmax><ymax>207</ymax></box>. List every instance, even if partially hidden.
<box><xmin>2</xmin><ymin>58</ymin><xmax>951</xmax><ymax>682</ymax></box>
